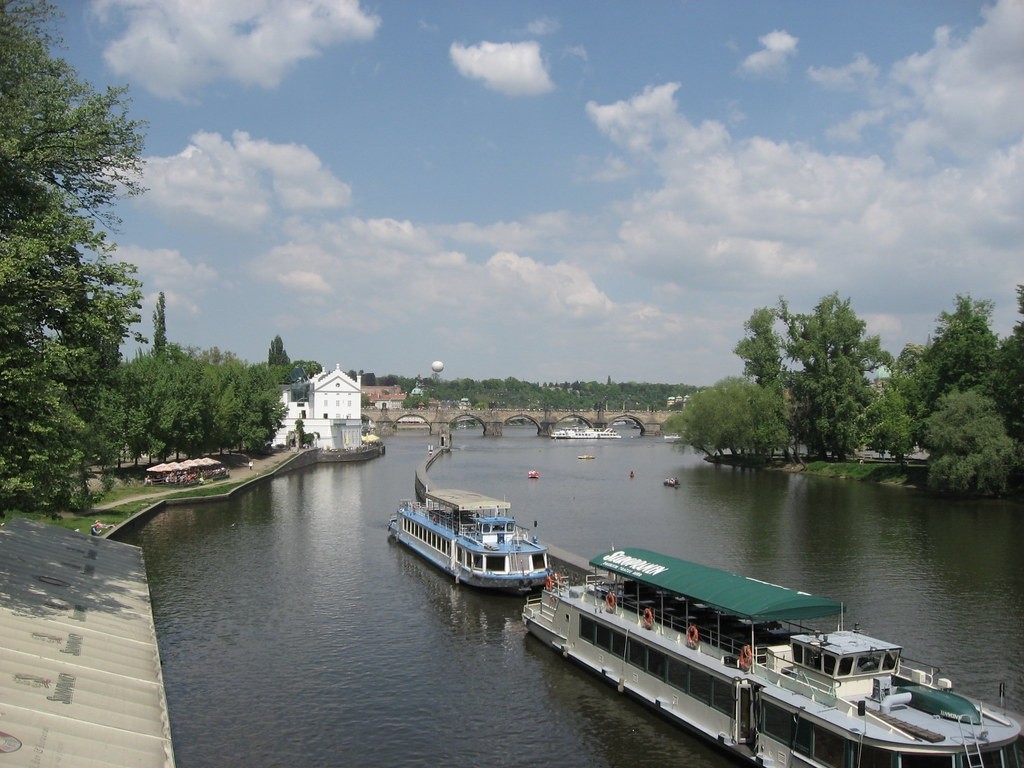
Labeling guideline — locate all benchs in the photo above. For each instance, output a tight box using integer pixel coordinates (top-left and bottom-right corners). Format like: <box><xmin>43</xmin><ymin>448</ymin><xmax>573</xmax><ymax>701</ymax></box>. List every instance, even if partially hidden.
<box><xmin>602</xmin><ymin>581</ymin><xmax>788</xmax><ymax>654</ymax></box>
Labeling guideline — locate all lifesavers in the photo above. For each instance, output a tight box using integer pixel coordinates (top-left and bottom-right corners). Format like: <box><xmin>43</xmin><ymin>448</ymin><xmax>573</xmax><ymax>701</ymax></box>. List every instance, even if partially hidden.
<box><xmin>606</xmin><ymin>593</ymin><xmax>614</xmax><ymax>609</ymax></box>
<box><xmin>546</xmin><ymin>577</ymin><xmax>552</xmax><ymax>590</ymax></box>
<box><xmin>642</xmin><ymin>609</ymin><xmax>653</xmax><ymax>628</ymax></box>
<box><xmin>433</xmin><ymin>516</ymin><xmax>437</xmax><ymax>523</ymax></box>
<box><xmin>686</xmin><ymin>626</ymin><xmax>698</xmax><ymax>648</ymax></box>
<box><xmin>740</xmin><ymin>647</ymin><xmax>752</xmax><ymax>670</ymax></box>
<box><xmin>551</xmin><ymin>574</ymin><xmax>561</xmax><ymax>585</ymax></box>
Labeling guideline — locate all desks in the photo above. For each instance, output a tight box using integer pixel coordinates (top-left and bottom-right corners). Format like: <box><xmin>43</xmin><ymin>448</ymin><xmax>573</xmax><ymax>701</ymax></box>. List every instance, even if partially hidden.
<box><xmin>617</xmin><ymin>594</ymin><xmax>636</xmax><ymax>606</ymax></box>
<box><xmin>715</xmin><ymin>633</ymin><xmax>745</xmax><ymax>648</ymax></box>
<box><xmin>656</xmin><ymin>591</ymin><xmax>670</xmax><ymax>597</ymax></box>
<box><xmin>693</xmin><ymin>603</ymin><xmax>711</xmax><ymax>614</ymax></box>
<box><xmin>674</xmin><ymin>616</ymin><xmax>697</xmax><ymax>629</ymax></box>
<box><xmin>768</xmin><ymin>629</ymin><xmax>796</xmax><ymax>643</ymax></box>
<box><xmin>714</xmin><ymin>611</ymin><xmax>735</xmax><ymax>624</ymax></box>
<box><xmin>703</xmin><ymin>624</ymin><xmax>717</xmax><ymax>638</ymax></box>
<box><xmin>675</xmin><ymin>597</ymin><xmax>690</xmax><ymax>606</ymax></box>
<box><xmin>656</xmin><ymin>607</ymin><xmax>676</xmax><ymax>623</ymax></box>
<box><xmin>632</xmin><ymin>600</ymin><xmax>655</xmax><ymax>606</ymax></box>
<box><xmin>738</xmin><ymin>619</ymin><xmax>770</xmax><ymax>637</ymax></box>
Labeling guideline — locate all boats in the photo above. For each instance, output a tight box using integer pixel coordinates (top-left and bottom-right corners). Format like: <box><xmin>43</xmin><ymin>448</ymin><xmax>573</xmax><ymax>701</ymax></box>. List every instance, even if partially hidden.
<box><xmin>518</xmin><ymin>543</ymin><xmax>1024</xmax><ymax>768</ymax></box>
<box><xmin>387</xmin><ymin>489</ymin><xmax>554</xmax><ymax>590</ymax></box>
<box><xmin>664</xmin><ymin>433</ymin><xmax>682</xmax><ymax>442</ymax></box>
<box><xmin>529</xmin><ymin>471</ymin><xmax>539</xmax><ymax>478</ymax></box>
<box><xmin>578</xmin><ymin>456</ymin><xmax>595</xmax><ymax>459</ymax></box>
<box><xmin>550</xmin><ymin>426</ymin><xmax>622</xmax><ymax>440</ymax></box>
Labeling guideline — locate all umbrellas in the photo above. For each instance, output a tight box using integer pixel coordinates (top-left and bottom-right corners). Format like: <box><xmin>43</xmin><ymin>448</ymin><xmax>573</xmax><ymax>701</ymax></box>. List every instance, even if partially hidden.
<box><xmin>345</xmin><ymin>432</ymin><xmax>380</xmax><ymax>452</ymax></box>
<box><xmin>146</xmin><ymin>457</ymin><xmax>222</xmax><ymax>482</ymax></box>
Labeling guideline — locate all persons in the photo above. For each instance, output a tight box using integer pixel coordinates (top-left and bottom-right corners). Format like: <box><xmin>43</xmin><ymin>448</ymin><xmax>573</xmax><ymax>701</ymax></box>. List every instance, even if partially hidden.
<box><xmin>432</xmin><ymin>515</ymin><xmax>439</xmax><ymax>525</ymax></box>
<box><xmin>145</xmin><ymin>465</ymin><xmax>231</xmax><ymax>486</ymax></box>
<box><xmin>90</xmin><ymin>524</ymin><xmax>101</xmax><ymax>537</ymax></box>
<box><xmin>533</xmin><ymin>535</ymin><xmax>539</xmax><ymax>544</ymax></box>
<box><xmin>249</xmin><ymin>460</ymin><xmax>253</xmax><ymax>470</ymax></box>
<box><xmin>91</xmin><ymin>519</ymin><xmax>116</xmax><ymax>528</ymax></box>
<box><xmin>453</xmin><ymin>526</ymin><xmax>459</xmax><ymax>537</ymax></box>
